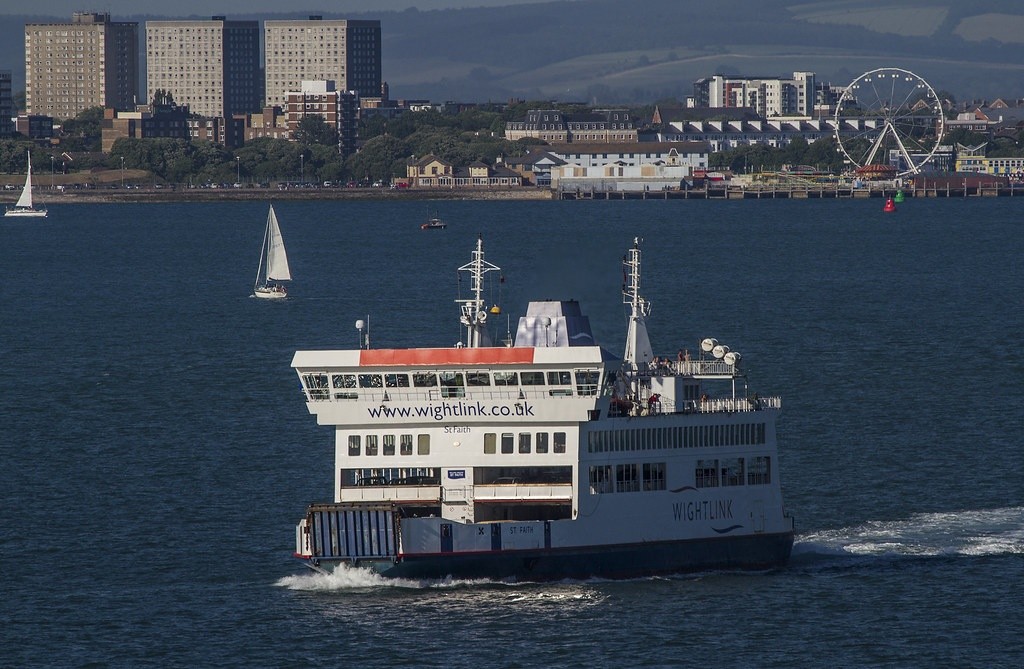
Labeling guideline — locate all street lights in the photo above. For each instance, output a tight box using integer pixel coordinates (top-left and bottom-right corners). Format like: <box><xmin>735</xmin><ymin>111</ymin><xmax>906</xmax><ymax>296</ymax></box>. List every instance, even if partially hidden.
<box><xmin>300</xmin><ymin>154</ymin><xmax>304</xmax><ymax>182</ymax></box>
<box><xmin>51</xmin><ymin>156</ymin><xmax>55</xmax><ymax>190</ymax></box>
<box><xmin>236</xmin><ymin>156</ymin><xmax>240</xmax><ymax>182</ymax></box>
<box><xmin>121</xmin><ymin>156</ymin><xmax>124</xmax><ymax>187</ymax></box>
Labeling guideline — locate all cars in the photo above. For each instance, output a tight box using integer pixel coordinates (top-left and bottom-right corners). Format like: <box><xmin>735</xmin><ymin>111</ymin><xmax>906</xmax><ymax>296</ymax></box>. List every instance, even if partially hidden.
<box><xmin>0</xmin><ymin>180</ymin><xmax>410</xmax><ymax>191</ymax></box>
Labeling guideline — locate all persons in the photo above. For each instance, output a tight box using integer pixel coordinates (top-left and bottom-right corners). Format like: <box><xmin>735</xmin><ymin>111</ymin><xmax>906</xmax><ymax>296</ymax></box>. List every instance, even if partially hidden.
<box><xmin>648</xmin><ymin>348</ymin><xmax>691</xmax><ymax>377</ymax></box>
<box><xmin>611</xmin><ymin>392</ymin><xmax>713</xmax><ymax>416</ymax></box>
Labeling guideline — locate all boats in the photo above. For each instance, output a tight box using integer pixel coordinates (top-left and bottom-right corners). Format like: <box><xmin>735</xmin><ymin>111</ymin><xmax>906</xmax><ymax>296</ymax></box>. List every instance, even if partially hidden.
<box><xmin>420</xmin><ymin>210</ymin><xmax>448</xmax><ymax>230</ymax></box>
<box><xmin>884</xmin><ymin>199</ymin><xmax>896</xmax><ymax>212</ymax></box>
<box><xmin>609</xmin><ymin>398</ymin><xmax>634</xmax><ymax>411</ymax></box>
<box><xmin>894</xmin><ymin>190</ymin><xmax>905</xmax><ymax>203</ymax></box>
<box><xmin>290</xmin><ymin>232</ymin><xmax>798</xmax><ymax>582</ymax></box>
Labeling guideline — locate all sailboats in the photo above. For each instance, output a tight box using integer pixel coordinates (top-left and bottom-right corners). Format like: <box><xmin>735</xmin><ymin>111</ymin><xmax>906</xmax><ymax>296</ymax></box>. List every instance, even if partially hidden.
<box><xmin>252</xmin><ymin>203</ymin><xmax>292</xmax><ymax>298</ymax></box>
<box><xmin>5</xmin><ymin>150</ymin><xmax>49</xmax><ymax>217</ymax></box>
